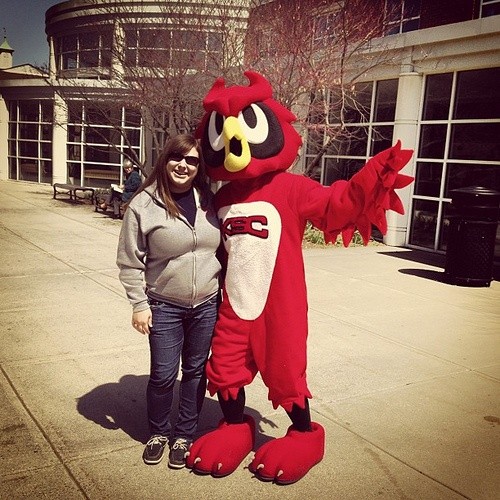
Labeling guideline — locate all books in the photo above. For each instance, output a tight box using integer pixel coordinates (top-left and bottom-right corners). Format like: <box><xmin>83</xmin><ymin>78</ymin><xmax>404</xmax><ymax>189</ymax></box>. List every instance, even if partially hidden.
<box><xmin>111</xmin><ymin>184</ymin><xmax>124</xmax><ymax>194</ymax></box>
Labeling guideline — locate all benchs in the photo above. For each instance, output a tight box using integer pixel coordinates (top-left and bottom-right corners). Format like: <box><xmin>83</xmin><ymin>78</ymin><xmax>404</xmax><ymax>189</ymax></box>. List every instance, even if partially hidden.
<box><xmin>53</xmin><ymin>183</ymin><xmax>94</xmax><ymax>205</ymax></box>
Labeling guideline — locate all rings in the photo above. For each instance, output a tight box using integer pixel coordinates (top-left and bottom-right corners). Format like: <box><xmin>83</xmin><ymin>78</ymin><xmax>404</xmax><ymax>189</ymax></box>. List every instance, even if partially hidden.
<box><xmin>134</xmin><ymin>322</ymin><xmax>137</xmax><ymax>324</ymax></box>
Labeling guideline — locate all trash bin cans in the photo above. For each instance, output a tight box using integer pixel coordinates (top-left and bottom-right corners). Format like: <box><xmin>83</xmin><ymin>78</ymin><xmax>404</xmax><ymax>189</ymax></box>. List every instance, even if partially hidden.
<box><xmin>444</xmin><ymin>185</ymin><xmax>500</xmax><ymax>287</ymax></box>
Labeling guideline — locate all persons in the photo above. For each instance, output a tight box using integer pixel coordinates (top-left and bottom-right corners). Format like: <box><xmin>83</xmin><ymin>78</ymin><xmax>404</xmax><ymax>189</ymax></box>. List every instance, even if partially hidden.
<box><xmin>118</xmin><ymin>133</ymin><xmax>222</xmax><ymax>469</ymax></box>
<box><xmin>96</xmin><ymin>159</ymin><xmax>142</xmax><ymax>219</ymax></box>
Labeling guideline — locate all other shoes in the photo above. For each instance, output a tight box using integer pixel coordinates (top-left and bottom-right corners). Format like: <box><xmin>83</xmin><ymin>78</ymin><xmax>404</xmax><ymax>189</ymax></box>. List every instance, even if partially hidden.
<box><xmin>95</xmin><ymin>204</ymin><xmax>107</xmax><ymax>211</ymax></box>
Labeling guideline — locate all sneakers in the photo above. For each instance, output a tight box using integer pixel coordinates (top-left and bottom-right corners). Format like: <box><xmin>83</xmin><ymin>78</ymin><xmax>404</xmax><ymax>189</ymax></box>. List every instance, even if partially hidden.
<box><xmin>143</xmin><ymin>432</ymin><xmax>169</xmax><ymax>464</ymax></box>
<box><xmin>168</xmin><ymin>435</ymin><xmax>193</xmax><ymax>468</ymax></box>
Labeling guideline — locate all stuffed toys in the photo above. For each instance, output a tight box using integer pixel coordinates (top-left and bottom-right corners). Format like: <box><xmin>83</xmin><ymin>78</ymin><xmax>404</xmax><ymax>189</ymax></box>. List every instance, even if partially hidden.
<box><xmin>185</xmin><ymin>71</ymin><xmax>416</xmax><ymax>484</ymax></box>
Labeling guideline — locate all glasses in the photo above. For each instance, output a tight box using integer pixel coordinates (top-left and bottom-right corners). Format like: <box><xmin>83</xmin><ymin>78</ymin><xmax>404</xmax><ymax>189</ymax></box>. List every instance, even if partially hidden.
<box><xmin>171</xmin><ymin>153</ymin><xmax>200</xmax><ymax>166</ymax></box>
